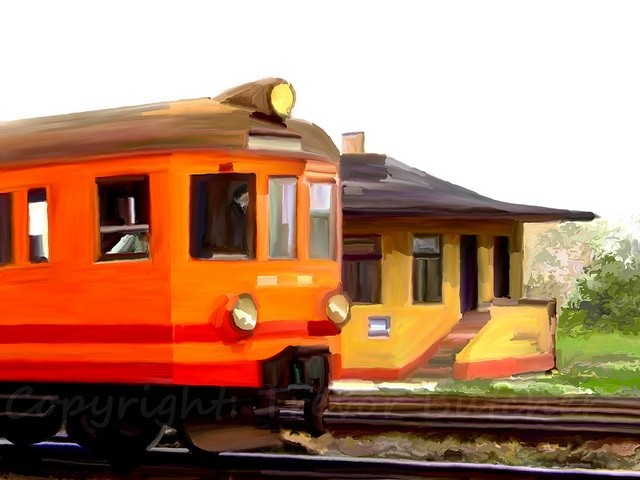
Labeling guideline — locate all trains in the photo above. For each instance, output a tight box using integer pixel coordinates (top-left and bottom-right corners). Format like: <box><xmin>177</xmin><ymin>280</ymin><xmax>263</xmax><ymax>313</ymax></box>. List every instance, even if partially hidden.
<box><xmin>0</xmin><ymin>78</ymin><xmax>353</xmax><ymax>458</ymax></box>
